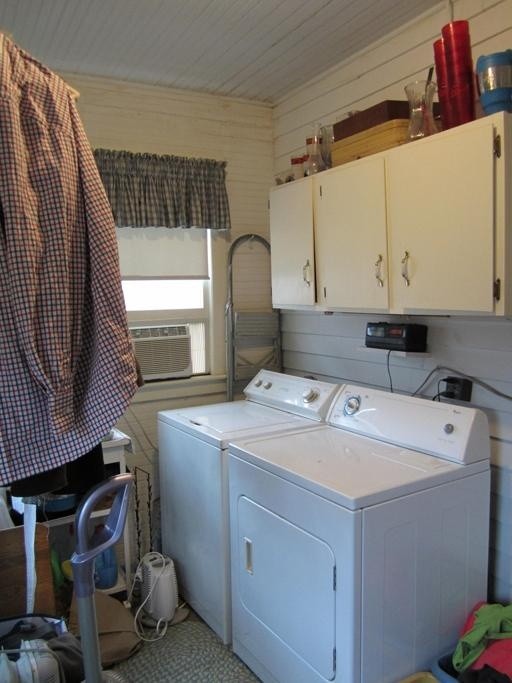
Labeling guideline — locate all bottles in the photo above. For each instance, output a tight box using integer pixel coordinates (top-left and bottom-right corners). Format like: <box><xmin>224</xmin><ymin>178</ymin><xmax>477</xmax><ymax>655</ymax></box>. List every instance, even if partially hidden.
<box><xmin>290</xmin><ymin>121</ymin><xmax>336</xmax><ymax>181</ymax></box>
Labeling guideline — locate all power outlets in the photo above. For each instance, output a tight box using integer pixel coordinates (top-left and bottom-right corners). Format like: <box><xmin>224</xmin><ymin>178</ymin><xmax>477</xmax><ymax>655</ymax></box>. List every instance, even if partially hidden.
<box><xmin>444</xmin><ymin>377</ymin><xmax>473</xmax><ymax>402</ymax></box>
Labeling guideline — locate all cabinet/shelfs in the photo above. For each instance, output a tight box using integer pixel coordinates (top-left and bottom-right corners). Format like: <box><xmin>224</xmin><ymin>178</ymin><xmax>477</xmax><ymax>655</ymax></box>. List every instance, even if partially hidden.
<box><xmin>269</xmin><ymin>174</ymin><xmax>316</xmax><ymax>315</ymax></box>
<box><xmin>47</xmin><ymin>426</ymin><xmax>137</xmax><ymax>617</ymax></box>
<box><xmin>312</xmin><ymin>110</ymin><xmax>509</xmax><ymax>317</ymax></box>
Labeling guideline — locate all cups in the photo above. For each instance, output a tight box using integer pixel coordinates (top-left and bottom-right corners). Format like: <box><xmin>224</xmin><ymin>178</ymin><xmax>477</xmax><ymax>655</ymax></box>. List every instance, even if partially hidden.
<box><xmin>404</xmin><ymin>20</ymin><xmax>477</xmax><ymax>143</ymax></box>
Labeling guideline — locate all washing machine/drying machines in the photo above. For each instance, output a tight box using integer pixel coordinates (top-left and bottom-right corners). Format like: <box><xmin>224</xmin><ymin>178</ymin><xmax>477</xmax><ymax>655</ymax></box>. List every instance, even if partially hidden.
<box><xmin>225</xmin><ymin>382</ymin><xmax>493</xmax><ymax>681</ymax></box>
<box><xmin>155</xmin><ymin>369</ymin><xmax>344</xmax><ymax>649</ymax></box>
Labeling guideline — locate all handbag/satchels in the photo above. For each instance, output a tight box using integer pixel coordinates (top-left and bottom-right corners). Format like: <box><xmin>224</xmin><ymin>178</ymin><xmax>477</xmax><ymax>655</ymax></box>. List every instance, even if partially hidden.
<box><xmin>1</xmin><ymin>614</ymin><xmax>70</xmax><ymax>660</ymax></box>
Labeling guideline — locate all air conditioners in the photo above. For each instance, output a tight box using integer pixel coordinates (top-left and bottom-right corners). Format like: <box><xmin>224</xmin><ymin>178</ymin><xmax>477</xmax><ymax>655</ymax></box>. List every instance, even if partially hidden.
<box><xmin>129</xmin><ymin>323</ymin><xmax>193</xmax><ymax>384</ymax></box>
